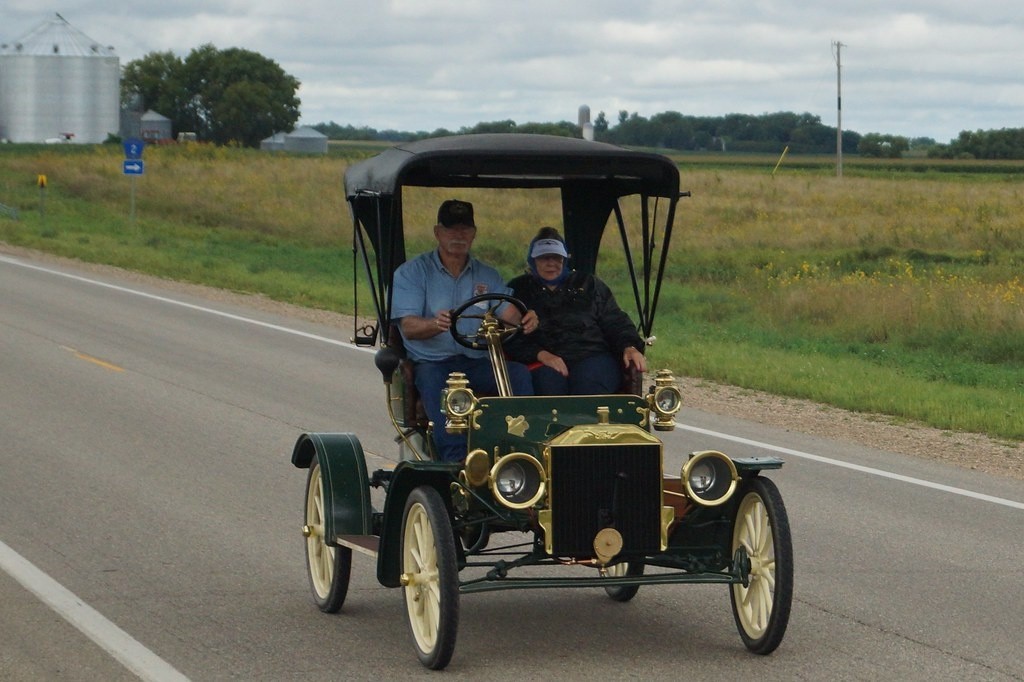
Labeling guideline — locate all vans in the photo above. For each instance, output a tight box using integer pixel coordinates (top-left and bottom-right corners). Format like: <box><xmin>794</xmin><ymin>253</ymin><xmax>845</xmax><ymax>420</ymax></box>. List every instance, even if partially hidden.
<box><xmin>142</xmin><ymin>130</ymin><xmax>177</xmax><ymax>145</ymax></box>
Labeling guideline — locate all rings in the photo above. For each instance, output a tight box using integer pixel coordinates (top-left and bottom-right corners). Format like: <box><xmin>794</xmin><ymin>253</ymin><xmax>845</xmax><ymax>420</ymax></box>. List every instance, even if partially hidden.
<box><xmin>436</xmin><ymin>321</ymin><xmax>439</xmax><ymax>325</ymax></box>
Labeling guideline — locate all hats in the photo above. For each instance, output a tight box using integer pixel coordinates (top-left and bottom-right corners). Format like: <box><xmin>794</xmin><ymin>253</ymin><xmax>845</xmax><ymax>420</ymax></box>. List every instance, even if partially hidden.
<box><xmin>437</xmin><ymin>199</ymin><xmax>474</xmax><ymax>228</ymax></box>
<box><xmin>531</xmin><ymin>239</ymin><xmax>568</xmax><ymax>258</ymax></box>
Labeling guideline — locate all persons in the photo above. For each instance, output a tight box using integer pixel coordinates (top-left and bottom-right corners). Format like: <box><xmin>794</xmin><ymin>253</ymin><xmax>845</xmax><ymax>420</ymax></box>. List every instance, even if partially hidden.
<box><xmin>390</xmin><ymin>200</ymin><xmax>540</xmax><ymax>470</ymax></box>
<box><xmin>498</xmin><ymin>227</ymin><xmax>648</xmax><ymax>395</ymax></box>
